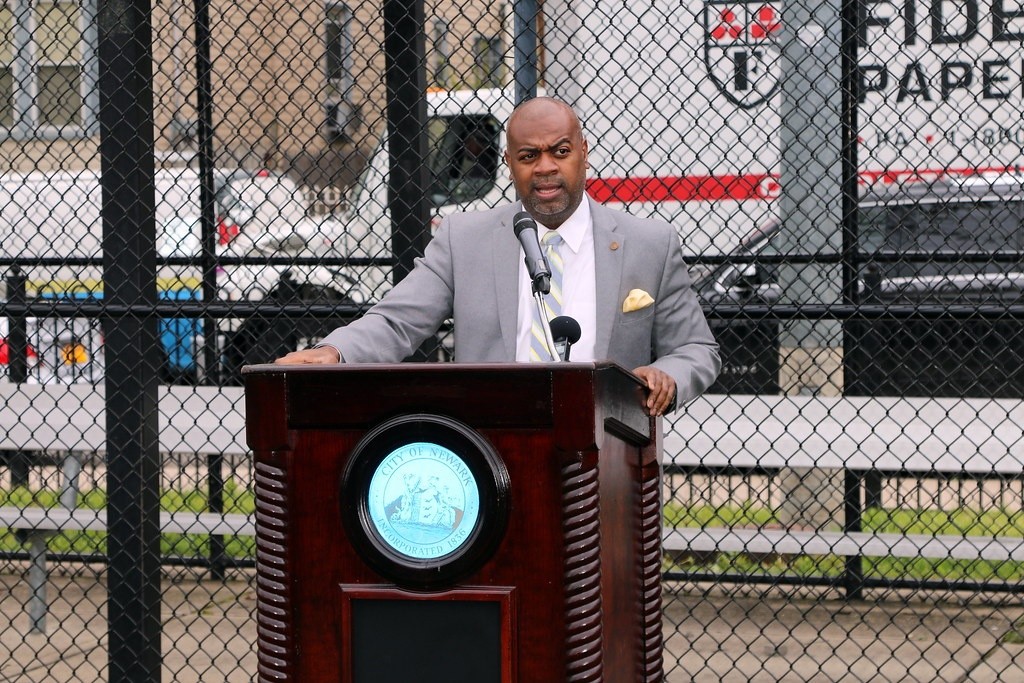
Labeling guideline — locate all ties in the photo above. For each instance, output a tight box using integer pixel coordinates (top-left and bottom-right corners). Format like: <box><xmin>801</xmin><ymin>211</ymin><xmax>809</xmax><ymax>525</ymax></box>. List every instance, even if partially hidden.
<box><xmin>529</xmin><ymin>232</ymin><xmax>564</xmax><ymax>363</ymax></box>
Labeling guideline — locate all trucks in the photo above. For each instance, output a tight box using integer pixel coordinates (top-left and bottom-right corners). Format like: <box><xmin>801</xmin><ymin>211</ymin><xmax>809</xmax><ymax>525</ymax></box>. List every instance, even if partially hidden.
<box><xmin>201</xmin><ymin>0</ymin><xmax>1024</xmax><ymax>400</ymax></box>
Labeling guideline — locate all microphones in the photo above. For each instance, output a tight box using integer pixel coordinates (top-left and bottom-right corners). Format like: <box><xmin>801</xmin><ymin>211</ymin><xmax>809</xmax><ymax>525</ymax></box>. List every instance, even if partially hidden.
<box><xmin>512</xmin><ymin>211</ymin><xmax>550</xmax><ymax>295</ymax></box>
<box><xmin>549</xmin><ymin>316</ymin><xmax>581</xmax><ymax>362</ymax></box>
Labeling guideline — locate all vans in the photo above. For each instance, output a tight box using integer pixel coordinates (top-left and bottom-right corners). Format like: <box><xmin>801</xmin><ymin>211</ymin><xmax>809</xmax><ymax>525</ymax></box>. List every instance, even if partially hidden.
<box><xmin>0</xmin><ymin>169</ymin><xmax>307</xmax><ymax>384</ymax></box>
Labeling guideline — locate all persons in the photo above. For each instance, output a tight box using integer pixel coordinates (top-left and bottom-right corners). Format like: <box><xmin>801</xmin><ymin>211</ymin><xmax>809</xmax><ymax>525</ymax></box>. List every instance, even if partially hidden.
<box><xmin>271</xmin><ymin>97</ymin><xmax>725</xmax><ymax>417</ymax></box>
<box><xmin>456</xmin><ymin>122</ymin><xmax>498</xmax><ymax>178</ymax></box>
<box><xmin>263</xmin><ymin>146</ymin><xmax>286</xmax><ymax>177</ymax></box>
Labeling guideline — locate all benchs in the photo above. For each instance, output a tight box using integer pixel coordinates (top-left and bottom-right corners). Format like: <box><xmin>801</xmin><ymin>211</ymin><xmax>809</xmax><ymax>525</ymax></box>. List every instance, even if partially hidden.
<box><xmin>0</xmin><ymin>382</ymin><xmax>1024</xmax><ymax>633</ymax></box>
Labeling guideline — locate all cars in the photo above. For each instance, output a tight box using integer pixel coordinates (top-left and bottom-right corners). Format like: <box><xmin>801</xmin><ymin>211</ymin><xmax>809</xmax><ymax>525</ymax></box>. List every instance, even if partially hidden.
<box><xmin>683</xmin><ymin>182</ymin><xmax>1024</xmax><ymax>397</ymax></box>
